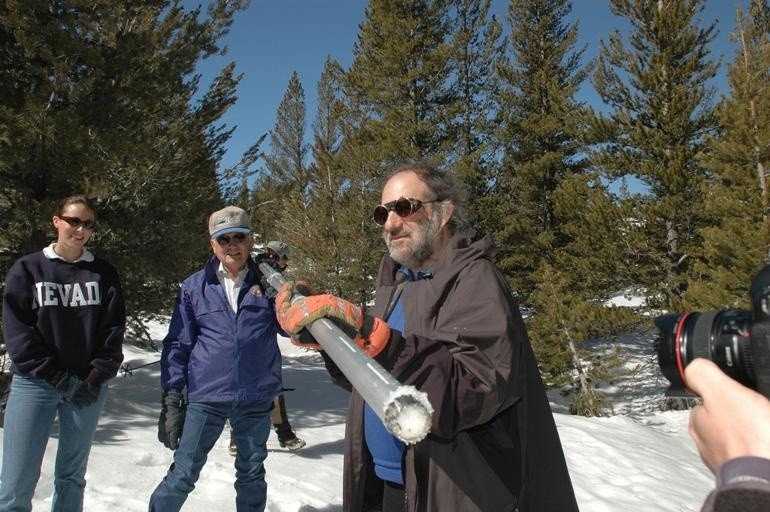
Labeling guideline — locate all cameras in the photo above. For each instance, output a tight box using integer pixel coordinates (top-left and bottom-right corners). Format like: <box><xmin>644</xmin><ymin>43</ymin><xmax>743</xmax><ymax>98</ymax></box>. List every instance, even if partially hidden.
<box><xmin>656</xmin><ymin>265</ymin><xmax>770</xmax><ymax>399</ymax></box>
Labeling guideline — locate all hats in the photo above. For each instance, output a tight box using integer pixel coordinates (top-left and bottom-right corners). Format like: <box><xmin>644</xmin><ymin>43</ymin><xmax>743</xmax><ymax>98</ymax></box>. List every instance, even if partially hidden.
<box><xmin>207</xmin><ymin>204</ymin><xmax>254</xmax><ymax>240</ymax></box>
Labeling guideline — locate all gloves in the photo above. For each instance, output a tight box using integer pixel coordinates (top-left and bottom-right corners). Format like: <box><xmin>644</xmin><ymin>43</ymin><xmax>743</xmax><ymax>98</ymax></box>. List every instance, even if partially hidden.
<box><xmin>274</xmin><ymin>279</ymin><xmax>391</xmax><ymax>358</ymax></box>
<box><xmin>158</xmin><ymin>392</ymin><xmax>185</xmax><ymax>450</ymax></box>
<box><xmin>54</xmin><ymin>366</ymin><xmax>108</xmax><ymax>410</ymax></box>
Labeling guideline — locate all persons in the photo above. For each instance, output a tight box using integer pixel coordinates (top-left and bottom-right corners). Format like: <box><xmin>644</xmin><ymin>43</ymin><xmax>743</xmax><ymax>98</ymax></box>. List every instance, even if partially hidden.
<box><xmin>684</xmin><ymin>357</ymin><xmax>770</xmax><ymax>512</ymax></box>
<box><xmin>0</xmin><ymin>194</ymin><xmax>127</xmax><ymax>512</ymax></box>
<box><xmin>148</xmin><ymin>205</ymin><xmax>290</xmax><ymax>512</ymax></box>
<box><xmin>230</xmin><ymin>240</ymin><xmax>306</xmax><ymax>456</ymax></box>
<box><xmin>274</xmin><ymin>164</ymin><xmax>580</xmax><ymax>512</ymax></box>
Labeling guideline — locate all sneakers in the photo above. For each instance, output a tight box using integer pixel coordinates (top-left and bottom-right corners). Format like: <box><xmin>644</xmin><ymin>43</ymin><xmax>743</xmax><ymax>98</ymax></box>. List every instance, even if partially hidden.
<box><xmin>277</xmin><ymin>436</ymin><xmax>308</xmax><ymax>452</ymax></box>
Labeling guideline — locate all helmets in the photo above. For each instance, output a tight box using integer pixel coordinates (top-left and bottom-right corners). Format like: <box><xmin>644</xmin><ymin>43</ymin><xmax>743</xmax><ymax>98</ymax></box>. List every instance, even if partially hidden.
<box><xmin>265</xmin><ymin>237</ymin><xmax>291</xmax><ymax>260</ymax></box>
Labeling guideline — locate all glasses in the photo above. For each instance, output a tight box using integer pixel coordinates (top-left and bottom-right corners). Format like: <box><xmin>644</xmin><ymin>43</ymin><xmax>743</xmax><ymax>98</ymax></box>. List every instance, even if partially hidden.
<box><xmin>371</xmin><ymin>197</ymin><xmax>439</xmax><ymax>225</ymax></box>
<box><xmin>212</xmin><ymin>234</ymin><xmax>248</xmax><ymax>246</ymax></box>
<box><xmin>57</xmin><ymin>213</ymin><xmax>97</xmax><ymax>231</ymax></box>
